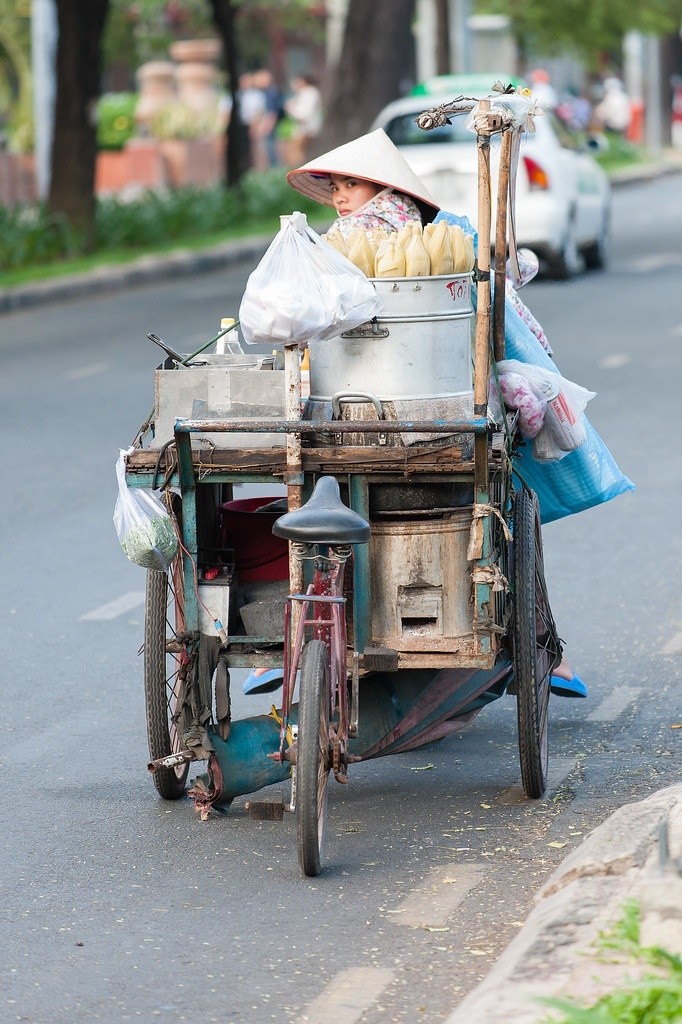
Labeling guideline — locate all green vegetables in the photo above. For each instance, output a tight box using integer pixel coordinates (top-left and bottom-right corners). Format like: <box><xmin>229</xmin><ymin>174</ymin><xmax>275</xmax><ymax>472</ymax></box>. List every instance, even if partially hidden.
<box><xmin>122</xmin><ymin>516</ymin><xmax>177</xmax><ymax>574</ymax></box>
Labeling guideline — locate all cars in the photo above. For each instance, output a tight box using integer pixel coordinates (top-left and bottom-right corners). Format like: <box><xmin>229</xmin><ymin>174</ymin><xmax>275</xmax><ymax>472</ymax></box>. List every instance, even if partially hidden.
<box><xmin>365</xmin><ymin>69</ymin><xmax>616</xmax><ymax>283</ymax></box>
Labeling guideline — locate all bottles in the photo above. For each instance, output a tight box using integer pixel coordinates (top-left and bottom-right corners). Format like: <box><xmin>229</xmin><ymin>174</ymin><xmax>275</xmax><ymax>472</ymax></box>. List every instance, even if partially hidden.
<box><xmin>300</xmin><ymin>349</ymin><xmax>310</xmax><ymax>407</ymax></box>
<box><xmin>212</xmin><ymin>318</ymin><xmax>246</xmax><ymax>354</ymax></box>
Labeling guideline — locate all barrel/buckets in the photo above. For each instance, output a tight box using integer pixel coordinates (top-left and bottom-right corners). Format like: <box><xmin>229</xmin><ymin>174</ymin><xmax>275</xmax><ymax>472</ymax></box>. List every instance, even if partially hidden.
<box><xmin>223</xmin><ymin>497</ymin><xmax>290</xmax><ymax>583</ymax></box>
<box><xmin>368</xmin><ymin>515</ymin><xmax>474</xmax><ymax>642</ymax></box>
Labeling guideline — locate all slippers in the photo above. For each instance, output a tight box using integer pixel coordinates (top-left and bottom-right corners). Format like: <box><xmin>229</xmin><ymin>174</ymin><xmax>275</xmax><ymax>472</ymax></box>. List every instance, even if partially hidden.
<box><xmin>242</xmin><ymin>669</ymin><xmax>284</xmax><ymax>695</ymax></box>
<box><xmin>549</xmin><ymin>672</ymin><xmax>590</xmax><ymax>698</ymax></box>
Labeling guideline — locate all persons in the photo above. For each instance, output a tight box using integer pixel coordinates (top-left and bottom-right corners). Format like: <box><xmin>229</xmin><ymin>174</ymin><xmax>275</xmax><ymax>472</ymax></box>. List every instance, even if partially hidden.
<box><xmin>286</xmin><ymin>128</ymin><xmax>587</xmax><ymax>699</ymax></box>
<box><xmin>221</xmin><ymin>70</ymin><xmax>324</xmax><ymax>167</ymax></box>
<box><xmin>531</xmin><ymin>67</ymin><xmax>630</xmax><ymax>139</ymax></box>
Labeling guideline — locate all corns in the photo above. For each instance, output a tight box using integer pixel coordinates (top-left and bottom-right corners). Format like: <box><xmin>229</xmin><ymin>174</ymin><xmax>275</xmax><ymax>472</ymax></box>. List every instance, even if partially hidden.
<box><xmin>319</xmin><ymin>220</ymin><xmax>475</xmax><ymax>276</ymax></box>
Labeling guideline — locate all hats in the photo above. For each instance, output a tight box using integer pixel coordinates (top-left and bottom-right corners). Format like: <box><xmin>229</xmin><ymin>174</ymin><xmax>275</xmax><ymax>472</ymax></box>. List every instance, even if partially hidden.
<box><xmin>286</xmin><ymin>127</ymin><xmax>440</xmax><ymax>227</ymax></box>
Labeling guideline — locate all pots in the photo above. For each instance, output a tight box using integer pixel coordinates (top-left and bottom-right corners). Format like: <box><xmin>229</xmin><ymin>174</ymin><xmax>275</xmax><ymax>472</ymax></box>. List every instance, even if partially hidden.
<box><xmin>301</xmin><ymin>271</ymin><xmax>474</xmax><ymax>402</ymax></box>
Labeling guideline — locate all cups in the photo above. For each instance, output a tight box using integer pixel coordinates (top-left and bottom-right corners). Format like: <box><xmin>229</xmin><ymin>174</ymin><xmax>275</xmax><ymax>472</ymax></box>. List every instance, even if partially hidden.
<box><xmin>470</xmin><ymin>276</ymin><xmax>616</xmax><ymax>518</ymax></box>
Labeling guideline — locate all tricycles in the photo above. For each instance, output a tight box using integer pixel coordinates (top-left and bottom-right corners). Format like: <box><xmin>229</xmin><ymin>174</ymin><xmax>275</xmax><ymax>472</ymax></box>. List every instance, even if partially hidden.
<box><xmin>106</xmin><ymin>78</ymin><xmax>566</xmax><ymax>881</ymax></box>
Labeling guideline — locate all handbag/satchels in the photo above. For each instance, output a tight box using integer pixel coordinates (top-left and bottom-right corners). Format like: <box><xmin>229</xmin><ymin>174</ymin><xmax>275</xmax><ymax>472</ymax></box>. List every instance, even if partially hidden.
<box><xmin>495</xmin><ymin>360</ymin><xmax>597</xmax><ymax>462</ymax></box>
<box><xmin>239</xmin><ymin>217</ymin><xmax>383</xmax><ymax>343</ymax></box>
<box><xmin>112</xmin><ymin>446</ymin><xmax>178</xmax><ymax>574</ymax></box>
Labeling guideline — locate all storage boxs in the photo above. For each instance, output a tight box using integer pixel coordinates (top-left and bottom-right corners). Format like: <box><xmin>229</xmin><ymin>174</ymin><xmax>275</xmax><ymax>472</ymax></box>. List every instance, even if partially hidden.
<box><xmin>155</xmin><ymin>354</ymin><xmax>288</xmax><ymax>449</ymax></box>
